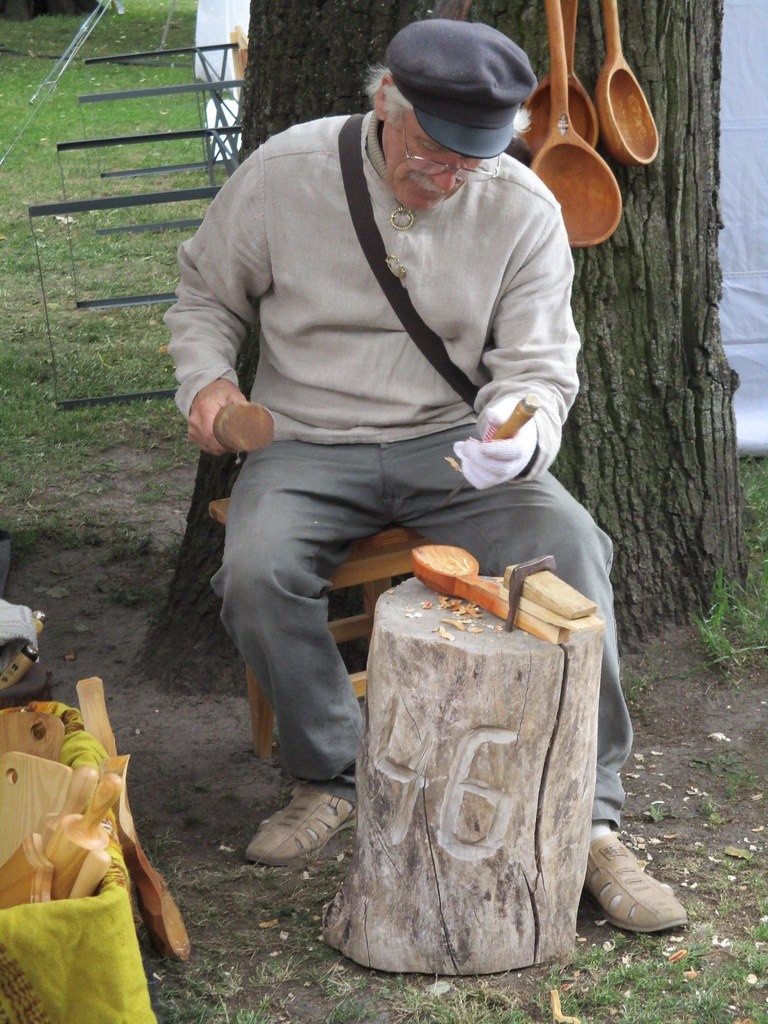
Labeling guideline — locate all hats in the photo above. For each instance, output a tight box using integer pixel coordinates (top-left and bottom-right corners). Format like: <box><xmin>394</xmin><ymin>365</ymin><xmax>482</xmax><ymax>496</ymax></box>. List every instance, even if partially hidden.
<box><xmin>385</xmin><ymin>18</ymin><xmax>538</xmax><ymax>159</ymax></box>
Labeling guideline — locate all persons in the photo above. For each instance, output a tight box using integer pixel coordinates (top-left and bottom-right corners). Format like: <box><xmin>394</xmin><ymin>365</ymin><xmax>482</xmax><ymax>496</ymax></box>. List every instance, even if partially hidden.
<box><xmin>160</xmin><ymin>19</ymin><xmax>691</xmax><ymax>934</ymax></box>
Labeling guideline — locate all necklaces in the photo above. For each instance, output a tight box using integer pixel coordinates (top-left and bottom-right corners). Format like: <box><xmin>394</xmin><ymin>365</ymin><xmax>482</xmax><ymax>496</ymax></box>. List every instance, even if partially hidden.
<box><xmin>384</xmin><ymin>204</ymin><xmax>414</xmax><ymax>277</ymax></box>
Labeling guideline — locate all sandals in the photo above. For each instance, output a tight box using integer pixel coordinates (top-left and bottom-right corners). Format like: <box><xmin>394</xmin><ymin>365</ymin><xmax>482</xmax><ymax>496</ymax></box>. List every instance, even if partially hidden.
<box><xmin>586</xmin><ymin>833</ymin><xmax>689</xmax><ymax>932</ymax></box>
<box><xmin>242</xmin><ymin>791</ymin><xmax>357</xmax><ymax>865</ymax></box>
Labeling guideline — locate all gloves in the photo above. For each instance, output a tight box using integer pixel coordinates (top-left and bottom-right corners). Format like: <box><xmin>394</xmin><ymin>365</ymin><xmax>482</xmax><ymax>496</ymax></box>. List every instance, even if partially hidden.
<box><xmin>459</xmin><ymin>397</ymin><xmax>536</xmax><ymax>491</ymax></box>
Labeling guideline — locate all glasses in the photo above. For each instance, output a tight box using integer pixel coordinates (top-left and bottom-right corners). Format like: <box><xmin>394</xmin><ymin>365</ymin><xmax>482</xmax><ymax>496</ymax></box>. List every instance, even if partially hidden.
<box><xmin>399</xmin><ymin>111</ymin><xmax>502</xmax><ymax>182</ymax></box>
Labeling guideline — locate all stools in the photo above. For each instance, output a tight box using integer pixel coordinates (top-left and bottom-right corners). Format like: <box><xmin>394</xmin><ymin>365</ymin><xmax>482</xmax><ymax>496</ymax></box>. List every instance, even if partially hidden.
<box><xmin>208</xmin><ymin>497</ymin><xmax>436</xmax><ymax>759</ymax></box>
